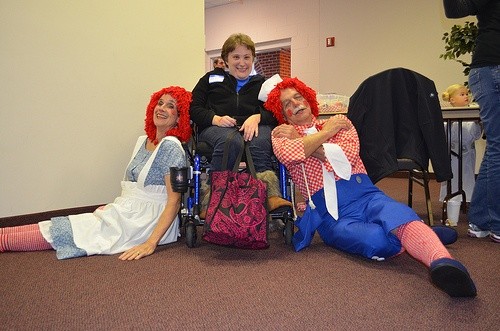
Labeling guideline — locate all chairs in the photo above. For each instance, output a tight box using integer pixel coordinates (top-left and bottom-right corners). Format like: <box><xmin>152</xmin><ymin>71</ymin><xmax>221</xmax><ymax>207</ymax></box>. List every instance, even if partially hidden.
<box><xmin>347</xmin><ymin>68</ymin><xmax>453</xmax><ymax>226</ymax></box>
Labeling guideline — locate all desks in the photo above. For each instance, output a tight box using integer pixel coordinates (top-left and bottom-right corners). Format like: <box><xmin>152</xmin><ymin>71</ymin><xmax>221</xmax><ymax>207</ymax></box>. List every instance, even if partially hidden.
<box><xmin>319</xmin><ymin>108</ymin><xmax>480</xmax><ymax>225</ymax></box>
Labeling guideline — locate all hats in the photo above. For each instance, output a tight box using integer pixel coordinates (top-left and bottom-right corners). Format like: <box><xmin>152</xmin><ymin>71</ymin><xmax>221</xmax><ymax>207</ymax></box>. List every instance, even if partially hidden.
<box><xmin>257</xmin><ymin>72</ymin><xmax>283</xmax><ymax>103</ymax></box>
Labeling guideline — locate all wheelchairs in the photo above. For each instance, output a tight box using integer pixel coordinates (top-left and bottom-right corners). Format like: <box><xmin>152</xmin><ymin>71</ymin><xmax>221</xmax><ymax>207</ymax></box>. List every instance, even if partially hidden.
<box><xmin>180</xmin><ymin>122</ymin><xmax>296</xmax><ymax>249</ymax></box>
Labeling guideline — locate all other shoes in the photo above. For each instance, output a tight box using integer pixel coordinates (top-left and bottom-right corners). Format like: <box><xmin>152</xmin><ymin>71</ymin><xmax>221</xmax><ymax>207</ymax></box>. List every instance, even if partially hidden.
<box><xmin>466</xmin><ymin>222</ymin><xmax>500</xmax><ymax>243</ymax></box>
<box><xmin>430</xmin><ymin>226</ymin><xmax>457</xmax><ymax>245</ymax></box>
<box><xmin>428</xmin><ymin>258</ymin><xmax>477</xmax><ymax>298</ymax></box>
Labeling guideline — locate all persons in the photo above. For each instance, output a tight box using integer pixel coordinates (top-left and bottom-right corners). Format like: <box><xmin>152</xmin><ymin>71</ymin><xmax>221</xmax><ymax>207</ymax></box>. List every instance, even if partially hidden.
<box><xmin>440</xmin><ymin>84</ymin><xmax>483</xmax><ymax>227</ymax></box>
<box><xmin>187</xmin><ymin>32</ymin><xmax>294</xmax><ymax>219</ymax></box>
<box><xmin>258</xmin><ymin>74</ymin><xmax>477</xmax><ymax>299</ymax></box>
<box><xmin>1</xmin><ymin>86</ymin><xmax>192</xmax><ymax>261</ymax></box>
<box><xmin>443</xmin><ymin>0</ymin><xmax>500</xmax><ymax>241</ymax></box>
<box><xmin>213</xmin><ymin>57</ymin><xmax>226</xmax><ymax>70</ymax></box>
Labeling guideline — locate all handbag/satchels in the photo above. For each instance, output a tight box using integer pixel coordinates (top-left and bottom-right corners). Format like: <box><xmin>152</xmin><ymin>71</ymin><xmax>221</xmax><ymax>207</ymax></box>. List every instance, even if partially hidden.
<box><xmin>201</xmin><ymin>128</ymin><xmax>270</xmax><ymax>250</ymax></box>
<box><xmin>291</xmin><ymin>204</ymin><xmax>323</xmax><ymax>252</ymax></box>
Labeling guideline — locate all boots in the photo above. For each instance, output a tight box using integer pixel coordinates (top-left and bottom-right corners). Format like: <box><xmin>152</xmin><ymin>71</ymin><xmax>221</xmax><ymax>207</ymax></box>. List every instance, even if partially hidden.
<box><xmin>255</xmin><ymin>170</ymin><xmax>293</xmax><ymax>212</ymax></box>
<box><xmin>198</xmin><ymin>171</ymin><xmax>212</xmax><ymax>219</ymax></box>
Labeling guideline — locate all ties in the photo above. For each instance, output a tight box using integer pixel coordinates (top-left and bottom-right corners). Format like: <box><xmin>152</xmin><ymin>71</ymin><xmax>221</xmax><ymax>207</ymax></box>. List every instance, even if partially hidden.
<box><xmin>304</xmin><ymin>125</ymin><xmax>352</xmax><ymax>221</ymax></box>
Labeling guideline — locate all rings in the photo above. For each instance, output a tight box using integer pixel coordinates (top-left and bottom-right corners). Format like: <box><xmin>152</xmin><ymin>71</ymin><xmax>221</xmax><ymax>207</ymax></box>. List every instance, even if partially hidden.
<box><xmin>136</xmin><ymin>252</ymin><xmax>139</xmax><ymax>255</ymax></box>
